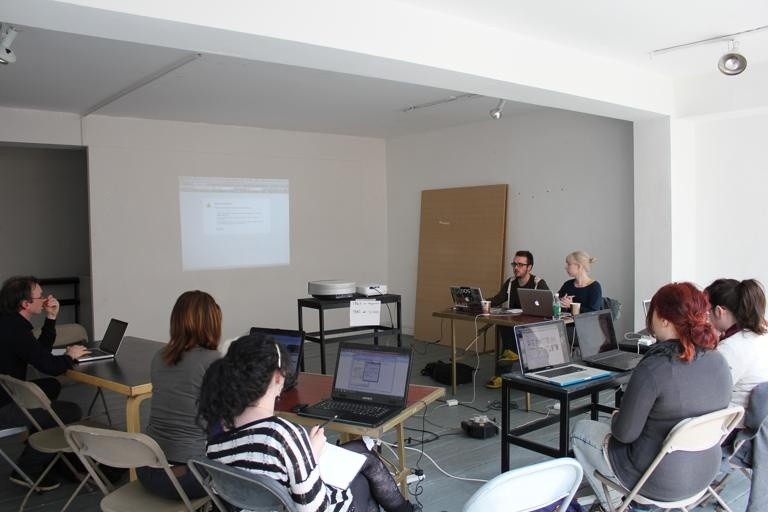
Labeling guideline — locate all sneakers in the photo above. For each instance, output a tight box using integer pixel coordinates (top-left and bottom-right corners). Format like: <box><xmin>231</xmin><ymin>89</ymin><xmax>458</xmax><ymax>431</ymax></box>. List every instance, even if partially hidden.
<box><xmin>485</xmin><ymin>376</ymin><xmax>502</xmax><ymax>388</ymax></box>
<box><xmin>498</xmin><ymin>349</ymin><xmax>519</xmax><ymax>365</ymax></box>
<box><xmin>9</xmin><ymin>471</ymin><xmax>61</xmax><ymax>491</ymax></box>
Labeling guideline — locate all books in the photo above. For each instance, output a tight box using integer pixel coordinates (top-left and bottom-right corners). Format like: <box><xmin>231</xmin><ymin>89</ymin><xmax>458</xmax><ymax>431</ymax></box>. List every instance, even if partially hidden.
<box><xmin>317</xmin><ymin>442</ymin><xmax>368</xmax><ymax>492</ymax></box>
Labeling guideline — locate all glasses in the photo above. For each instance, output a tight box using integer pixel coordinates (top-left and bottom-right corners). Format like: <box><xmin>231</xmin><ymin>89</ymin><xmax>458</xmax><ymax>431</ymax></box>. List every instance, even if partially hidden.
<box><xmin>27</xmin><ymin>292</ymin><xmax>45</xmax><ymax>301</ymax></box>
<box><xmin>511</xmin><ymin>263</ymin><xmax>530</xmax><ymax>268</ymax></box>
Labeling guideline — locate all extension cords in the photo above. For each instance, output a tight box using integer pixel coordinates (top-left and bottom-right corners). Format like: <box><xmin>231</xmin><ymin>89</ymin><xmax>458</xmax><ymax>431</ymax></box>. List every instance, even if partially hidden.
<box><xmin>397</xmin><ymin>474</ymin><xmax>425</xmax><ymax>487</ymax></box>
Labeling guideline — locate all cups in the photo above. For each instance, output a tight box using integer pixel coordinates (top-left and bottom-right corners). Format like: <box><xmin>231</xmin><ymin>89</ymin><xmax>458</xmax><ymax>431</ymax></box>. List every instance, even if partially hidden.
<box><xmin>568</xmin><ymin>302</ymin><xmax>581</xmax><ymax>316</ymax></box>
<box><xmin>480</xmin><ymin>300</ymin><xmax>491</xmax><ymax>314</ymax></box>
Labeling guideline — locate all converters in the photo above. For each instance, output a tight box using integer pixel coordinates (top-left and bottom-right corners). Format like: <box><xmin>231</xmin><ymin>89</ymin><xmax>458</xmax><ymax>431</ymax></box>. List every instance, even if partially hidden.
<box><xmin>638</xmin><ymin>338</ymin><xmax>652</xmax><ymax>346</ymax></box>
<box><xmin>446</xmin><ymin>399</ymin><xmax>458</xmax><ymax>406</ymax></box>
<box><xmin>554</xmin><ymin>403</ymin><xmax>560</xmax><ymax>410</ymax></box>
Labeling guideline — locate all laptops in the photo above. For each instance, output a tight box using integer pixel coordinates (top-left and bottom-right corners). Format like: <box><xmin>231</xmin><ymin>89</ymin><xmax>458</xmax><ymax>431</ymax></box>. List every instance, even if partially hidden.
<box><xmin>297</xmin><ymin>341</ymin><xmax>415</xmax><ymax>428</ymax></box>
<box><xmin>517</xmin><ymin>288</ymin><xmax>554</xmax><ymax>317</ymax></box>
<box><xmin>574</xmin><ymin>308</ymin><xmax>644</xmax><ymax>372</ymax></box>
<box><xmin>514</xmin><ymin>319</ymin><xmax>611</xmax><ymax>387</ymax></box>
<box><xmin>449</xmin><ymin>286</ymin><xmax>483</xmax><ymax>312</ymax></box>
<box><xmin>249</xmin><ymin>328</ymin><xmax>305</xmax><ymax>392</ymax></box>
<box><xmin>642</xmin><ymin>299</ymin><xmax>651</xmax><ymax>318</ymax></box>
<box><xmin>75</xmin><ymin>318</ymin><xmax>128</xmax><ymax>362</ymax></box>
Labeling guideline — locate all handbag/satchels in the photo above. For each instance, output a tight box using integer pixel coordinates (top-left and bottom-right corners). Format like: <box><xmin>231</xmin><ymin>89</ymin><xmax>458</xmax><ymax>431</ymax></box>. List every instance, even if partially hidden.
<box><xmin>421</xmin><ymin>359</ymin><xmax>480</xmax><ymax>385</ymax></box>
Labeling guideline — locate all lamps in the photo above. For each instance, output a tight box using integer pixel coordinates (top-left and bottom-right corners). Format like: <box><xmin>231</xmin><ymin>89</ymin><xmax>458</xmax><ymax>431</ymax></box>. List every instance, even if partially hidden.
<box><xmin>0</xmin><ymin>28</ymin><xmax>22</xmax><ymax>66</ymax></box>
<box><xmin>487</xmin><ymin>98</ymin><xmax>508</xmax><ymax>121</ymax></box>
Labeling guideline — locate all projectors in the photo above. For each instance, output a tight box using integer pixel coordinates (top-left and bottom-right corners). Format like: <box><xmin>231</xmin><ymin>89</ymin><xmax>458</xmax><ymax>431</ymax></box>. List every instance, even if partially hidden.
<box><xmin>357</xmin><ymin>282</ymin><xmax>388</xmax><ymax>297</ymax></box>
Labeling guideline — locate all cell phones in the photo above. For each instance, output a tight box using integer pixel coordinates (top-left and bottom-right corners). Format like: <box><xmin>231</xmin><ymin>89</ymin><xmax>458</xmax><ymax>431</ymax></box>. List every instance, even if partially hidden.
<box><xmin>290</xmin><ymin>403</ymin><xmax>308</xmax><ymax>413</ymax></box>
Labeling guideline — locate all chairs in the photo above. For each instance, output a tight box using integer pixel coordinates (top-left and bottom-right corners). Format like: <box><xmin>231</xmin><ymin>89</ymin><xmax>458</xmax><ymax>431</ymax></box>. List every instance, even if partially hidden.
<box><xmin>459</xmin><ymin>457</ymin><xmax>584</xmax><ymax>512</ymax></box>
<box><xmin>594</xmin><ymin>404</ymin><xmax>748</xmax><ymax>512</ymax></box>
<box><xmin>698</xmin><ymin>384</ymin><xmax>768</xmax><ymax>512</ymax></box>
<box><xmin>570</xmin><ymin>297</ymin><xmax>622</xmax><ymax>361</ymax></box>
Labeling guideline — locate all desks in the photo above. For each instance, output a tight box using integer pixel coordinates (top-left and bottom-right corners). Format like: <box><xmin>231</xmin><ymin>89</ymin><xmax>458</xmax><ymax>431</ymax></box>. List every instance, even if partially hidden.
<box><xmin>298</xmin><ymin>292</ymin><xmax>403</xmax><ymax>377</ymax></box>
<box><xmin>502</xmin><ymin>350</ymin><xmax>643</xmax><ymax>473</ymax></box>
<box><xmin>613</xmin><ymin>325</ymin><xmax>660</xmax><ymax>407</ymax></box>
<box><xmin>267</xmin><ymin>367</ymin><xmax>446</xmax><ymax>512</ymax></box>
<box><xmin>429</xmin><ymin>300</ymin><xmax>584</xmax><ymax>411</ymax></box>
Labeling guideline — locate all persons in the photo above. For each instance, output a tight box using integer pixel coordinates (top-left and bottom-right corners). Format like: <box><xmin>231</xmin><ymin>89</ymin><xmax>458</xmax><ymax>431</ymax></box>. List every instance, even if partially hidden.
<box><xmin>701</xmin><ymin>277</ymin><xmax>767</xmax><ymax>488</ymax></box>
<box><xmin>569</xmin><ymin>281</ymin><xmax>733</xmax><ymax>512</ymax></box>
<box><xmin>192</xmin><ymin>333</ymin><xmax>420</xmax><ymax>512</ymax></box>
<box><xmin>555</xmin><ymin>250</ymin><xmax>604</xmax><ymax>349</ymax></box>
<box><xmin>137</xmin><ymin>290</ymin><xmax>226</xmax><ymax>500</ymax></box>
<box><xmin>483</xmin><ymin>248</ymin><xmax>552</xmax><ymax>391</ymax></box>
<box><xmin>1</xmin><ymin>274</ymin><xmax>94</xmax><ymax>492</ymax></box>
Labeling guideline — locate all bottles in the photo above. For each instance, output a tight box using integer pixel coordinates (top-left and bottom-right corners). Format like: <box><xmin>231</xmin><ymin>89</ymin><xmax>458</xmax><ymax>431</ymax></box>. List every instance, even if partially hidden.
<box><xmin>551</xmin><ymin>291</ymin><xmax>561</xmax><ymax>322</ymax></box>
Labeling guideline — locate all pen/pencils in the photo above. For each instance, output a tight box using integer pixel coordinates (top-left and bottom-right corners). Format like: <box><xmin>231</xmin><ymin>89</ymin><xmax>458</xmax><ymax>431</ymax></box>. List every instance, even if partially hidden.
<box><xmin>318</xmin><ymin>414</ymin><xmax>338</xmax><ymax>431</ymax></box>
